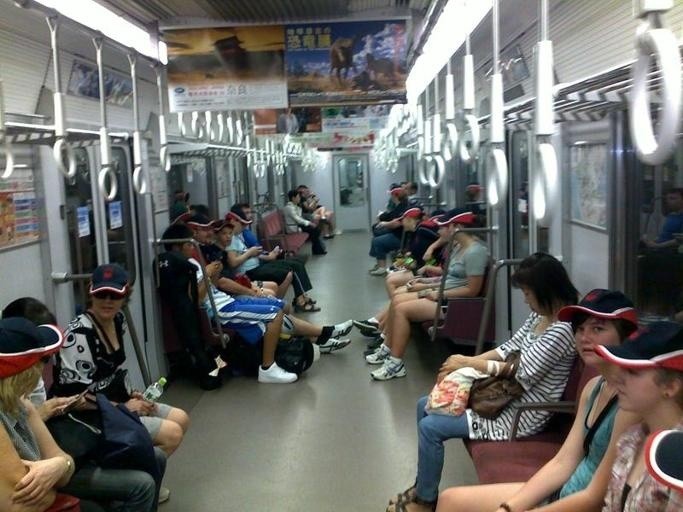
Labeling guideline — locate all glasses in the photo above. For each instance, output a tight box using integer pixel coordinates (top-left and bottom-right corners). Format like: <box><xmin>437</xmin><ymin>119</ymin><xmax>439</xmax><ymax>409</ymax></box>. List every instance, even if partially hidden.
<box><xmin>92</xmin><ymin>291</ymin><xmax>127</xmax><ymax>301</ymax></box>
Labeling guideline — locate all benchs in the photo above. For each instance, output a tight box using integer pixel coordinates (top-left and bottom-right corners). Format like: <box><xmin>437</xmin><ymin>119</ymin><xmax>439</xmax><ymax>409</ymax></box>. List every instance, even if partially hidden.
<box><xmin>453</xmin><ymin>345</ymin><xmax>606</xmax><ymax>492</ymax></box>
<box><xmin>150</xmin><ymin>252</ymin><xmax>242</xmax><ymax>356</ymax></box>
<box><xmin>255</xmin><ymin>203</ymin><xmax>312</xmax><ymax>257</ymax></box>
<box><xmin>416</xmin><ymin>252</ymin><xmax>496</xmax><ymax>346</ymax></box>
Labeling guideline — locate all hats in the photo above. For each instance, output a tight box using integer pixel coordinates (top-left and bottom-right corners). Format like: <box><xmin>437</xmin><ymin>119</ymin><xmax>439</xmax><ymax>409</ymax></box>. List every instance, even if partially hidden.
<box><xmin>595</xmin><ymin>319</ymin><xmax>683</xmax><ymax>375</ymax></box>
<box><xmin>170</xmin><ymin>202</ymin><xmax>190</xmax><ymax>225</ymax></box>
<box><xmin>225</xmin><ymin>207</ymin><xmax>253</xmax><ymax>226</ymax></box>
<box><xmin>88</xmin><ymin>263</ymin><xmax>132</xmax><ymax>294</ymax></box>
<box><xmin>391</xmin><ymin>188</ymin><xmax>475</xmax><ymax>226</ymax></box>
<box><xmin>187</xmin><ymin>212</ymin><xmax>216</xmax><ymax>228</ymax></box>
<box><xmin>644</xmin><ymin>426</ymin><xmax>683</xmax><ymax>495</ymax></box>
<box><xmin>467</xmin><ymin>185</ymin><xmax>484</xmax><ymax>193</ymax></box>
<box><xmin>0</xmin><ymin>316</ymin><xmax>66</xmax><ymax>379</ymax></box>
<box><xmin>557</xmin><ymin>288</ymin><xmax>640</xmax><ymax>328</ymax></box>
<box><xmin>214</xmin><ymin>217</ymin><xmax>235</xmax><ymax>235</ymax></box>
<box><xmin>190</xmin><ymin>204</ymin><xmax>214</xmax><ymax>220</ymax></box>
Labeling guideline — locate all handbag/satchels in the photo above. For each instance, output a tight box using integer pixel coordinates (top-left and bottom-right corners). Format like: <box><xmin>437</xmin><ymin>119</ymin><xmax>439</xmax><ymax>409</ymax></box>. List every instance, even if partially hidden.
<box><xmin>425</xmin><ymin>360</ymin><xmax>500</xmax><ymax>416</ymax></box>
<box><xmin>45</xmin><ymin>380</ymin><xmax>110</xmax><ymax>468</ymax></box>
<box><xmin>466</xmin><ymin>349</ymin><xmax>524</xmax><ymax>421</ymax></box>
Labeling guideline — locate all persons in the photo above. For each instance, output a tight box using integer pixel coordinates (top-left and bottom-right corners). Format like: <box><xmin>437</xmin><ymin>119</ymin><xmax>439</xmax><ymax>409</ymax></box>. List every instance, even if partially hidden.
<box><xmin>386</xmin><ymin>251</ymin><xmax>683</xmax><ymax>511</ymax></box>
<box><xmin>278</xmin><ymin>109</ymin><xmax>300</xmax><ymax>133</ymax></box>
<box><xmin>361</xmin><ymin>34</ymin><xmax>374</xmax><ymax>63</ymax></box>
<box><xmin>353</xmin><ymin>184</ymin><xmax>489</xmax><ymax>381</ymax></box>
<box><xmin>163</xmin><ymin>184</ymin><xmax>353</xmax><ymax>384</ymax></box>
<box><xmin>0</xmin><ymin>265</ymin><xmax>189</xmax><ymax>510</ymax></box>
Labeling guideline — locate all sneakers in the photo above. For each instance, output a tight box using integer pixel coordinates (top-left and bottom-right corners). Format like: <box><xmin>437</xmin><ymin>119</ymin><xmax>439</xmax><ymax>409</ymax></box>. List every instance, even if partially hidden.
<box><xmin>321</xmin><ymin>319</ymin><xmax>354</xmax><ymax>340</ymax></box>
<box><xmin>316</xmin><ymin>335</ymin><xmax>352</xmax><ymax>353</ymax></box>
<box><xmin>353</xmin><ymin>319</ymin><xmax>384</xmax><ymax>356</ymax></box>
<box><xmin>257</xmin><ymin>360</ymin><xmax>298</xmax><ymax>385</ymax></box>
<box><xmin>366</xmin><ymin>344</ymin><xmax>391</xmax><ymax>364</ymax></box>
<box><xmin>371</xmin><ymin>356</ymin><xmax>407</xmax><ymax>381</ymax></box>
<box><xmin>370</xmin><ymin>266</ymin><xmax>387</xmax><ymax>277</ymax></box>
<box><xmin>158</xmin><ymin>486</ymin><xmax>170</xmax><ymax>505</ymax></box>
<box><xmin>368</xmin><ymin>265</ymin><xmax>379</xmax><ymax>273</ymax></box>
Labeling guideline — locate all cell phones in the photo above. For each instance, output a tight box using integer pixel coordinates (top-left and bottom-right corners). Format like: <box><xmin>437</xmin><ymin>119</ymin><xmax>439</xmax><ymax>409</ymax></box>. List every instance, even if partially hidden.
<box><xmin>407</xmin><ymin>284</ymin><xmax>412</xmax><ymax>287</ymax></box>
<box><xmin>63</xmin><ymin>389</ymin><xmax>89</xmax><ymax>412</ymax></box>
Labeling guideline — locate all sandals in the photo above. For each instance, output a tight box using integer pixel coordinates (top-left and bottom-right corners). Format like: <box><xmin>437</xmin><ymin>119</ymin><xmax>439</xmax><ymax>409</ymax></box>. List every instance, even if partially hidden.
<box><xmin>291</xmin><ymin>296</ymin><xmax>317</xmax><ymax>306</ymax></box>
<box><xmin>385</xmin><ymin>496</ymin><xmax>437</xmax><ymax>512</ymax></box>
<box><xmin>295</xmin><ymin>301</ymin><xmax>321</xmax><ymax>313</ymax></box>
<box><xmin>389</xmin><ymin>479</ymin><xmax>418</xmax><ymax>506</ymax></box>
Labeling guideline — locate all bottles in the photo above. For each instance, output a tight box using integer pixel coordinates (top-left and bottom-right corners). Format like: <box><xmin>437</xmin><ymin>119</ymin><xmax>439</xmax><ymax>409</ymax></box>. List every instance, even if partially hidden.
<box><xmin>138</xmin><ymin>376</ymin><xmax>168</xmax><ymax>404</ymax></box>
